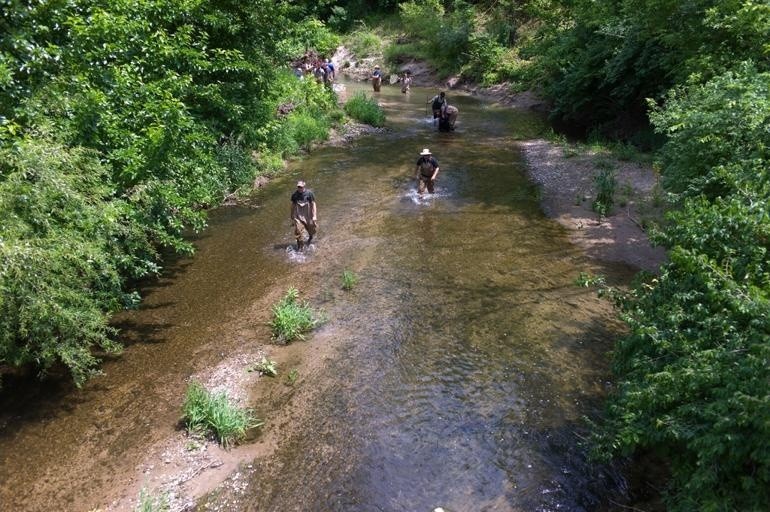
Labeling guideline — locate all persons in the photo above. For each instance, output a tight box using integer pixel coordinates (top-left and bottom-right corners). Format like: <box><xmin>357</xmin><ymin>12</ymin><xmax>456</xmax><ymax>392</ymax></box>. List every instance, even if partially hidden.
<box><xmin>397</xmin><ymin>70</ymin><xmax>412</xmax><ymax>94</ymax></box>
<box><xmin>440</xmin><ymin>104</ymin><xmax>458</xmax><ymax>131</ymax></box>
<box><xmin>290</xmin><ymin>181</ymin><xmax>316</xmax><ymax>252</ymax></box>
<box><xmin>413</xmin><ymin>148</ymin><xmax>440</xmax><ymax>199</ymax></box>
<box><xmin>423</xmin><ymin>92</ymin><xmax>446</xmax><ymax>127</ymax></box>
<box><xmin>296</xmin><ymin>58</ymin><xmax>335</xmax><ymax>83</ymax></box>
<box><xmin>371</xmin><ymin>65</ymin><xmax>381</xmax><ymax>92</ymax></box>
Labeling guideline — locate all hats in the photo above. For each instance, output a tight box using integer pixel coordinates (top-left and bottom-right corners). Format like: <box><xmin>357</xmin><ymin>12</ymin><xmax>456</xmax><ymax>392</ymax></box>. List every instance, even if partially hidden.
<box><xmin>296</xmin><ymin>180</ymin><xmax>308</xmax><ymax>188</ymax></box>
<box><xmin>373</xmin><ymin>64</ymin><xmax>381</xmax><ymax>70</ymax></box>
<box><xmin>419</xmin><ymin>148</ymin><xmax>433</xmax><ymax>156</ymax></box>
